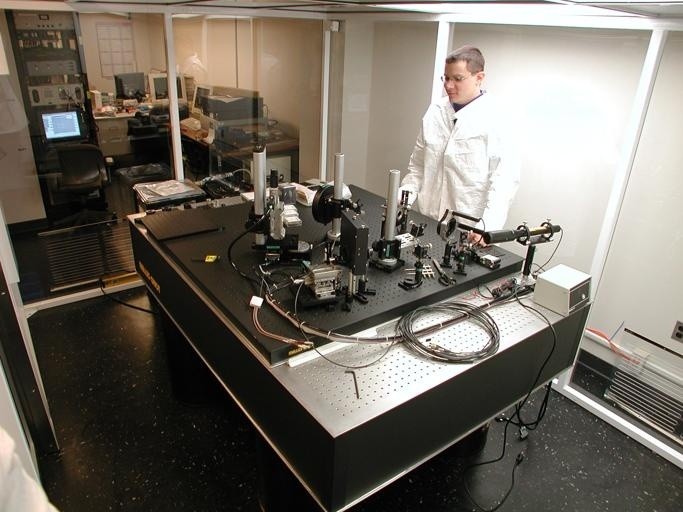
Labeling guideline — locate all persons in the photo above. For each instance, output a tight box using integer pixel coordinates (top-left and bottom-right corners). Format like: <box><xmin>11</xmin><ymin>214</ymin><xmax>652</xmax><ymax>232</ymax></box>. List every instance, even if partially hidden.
<box><xmin>397</xmin><ymin>45</ymin><xmax>518</xmax><ymax>249</ymax></box>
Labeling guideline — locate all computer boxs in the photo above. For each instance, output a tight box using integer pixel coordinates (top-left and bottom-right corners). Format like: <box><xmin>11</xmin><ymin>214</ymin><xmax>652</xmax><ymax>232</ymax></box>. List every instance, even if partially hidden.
<box><xmin>202</xmin><ymin>95</ymin><xmax>263</xmax><ymax>121</ymax></box>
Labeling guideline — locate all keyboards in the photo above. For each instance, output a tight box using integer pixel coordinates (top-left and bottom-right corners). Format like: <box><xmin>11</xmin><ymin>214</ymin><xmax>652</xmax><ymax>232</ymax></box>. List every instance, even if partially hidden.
<box><xmin>180</xmin><ymin>117</ymin><xmax>201</xmax><ymax>131</ymax></box>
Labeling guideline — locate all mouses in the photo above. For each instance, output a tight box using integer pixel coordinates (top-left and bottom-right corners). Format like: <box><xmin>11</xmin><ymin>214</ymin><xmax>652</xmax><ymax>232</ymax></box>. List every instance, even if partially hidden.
<box><xmin>203</xmin><ymin>132</ymin><xmax>208</xmax><ymax>138</ymax></box>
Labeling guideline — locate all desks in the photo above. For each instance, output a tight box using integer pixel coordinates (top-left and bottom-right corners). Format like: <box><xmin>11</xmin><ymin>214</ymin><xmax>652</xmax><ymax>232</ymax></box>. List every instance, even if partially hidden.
<box><xmin>92</xmin><ymin>102</ymin><xmax>298</xmax><ymax>189</ymax></box>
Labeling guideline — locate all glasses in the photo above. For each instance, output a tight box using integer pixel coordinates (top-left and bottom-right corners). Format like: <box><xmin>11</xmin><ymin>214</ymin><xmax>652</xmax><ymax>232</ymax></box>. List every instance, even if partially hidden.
<box><xmin>441</xmin><ymin>69</ymin><xmax>483</xmax><ymax>84</ymax></box>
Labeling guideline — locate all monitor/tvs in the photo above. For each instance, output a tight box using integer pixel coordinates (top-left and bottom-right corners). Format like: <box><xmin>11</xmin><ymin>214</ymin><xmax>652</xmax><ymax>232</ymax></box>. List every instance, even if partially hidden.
<box><xmin>115</xmin><ymin>72</ymin><xmax>145</xmax><ymax>103</ymax></box>
<box><xmin>41</xmin><ymin>110</ymin><xmax>82</xmax><ymax>140</ymax></box>
<box><xmin>192</xmin><ymin>85</ymin><xmax>213</xmax><ymax>114</ymax></box>
<box><xmin>148</xmin><ymin>73</ymin><xmax>188</xmax><ymax>106</ymax></box>
